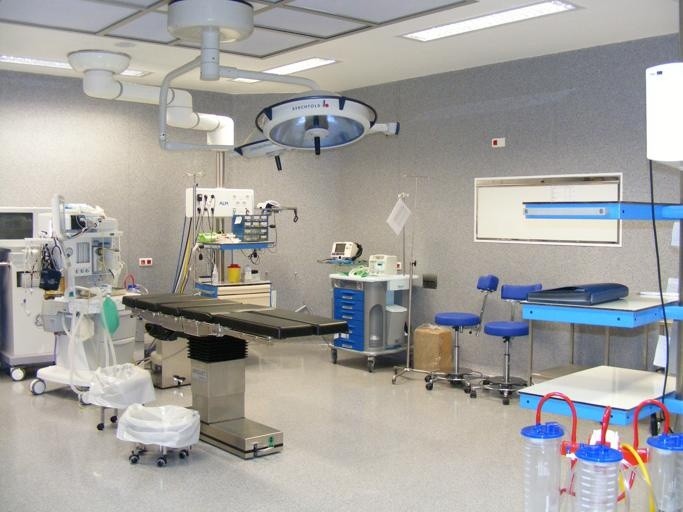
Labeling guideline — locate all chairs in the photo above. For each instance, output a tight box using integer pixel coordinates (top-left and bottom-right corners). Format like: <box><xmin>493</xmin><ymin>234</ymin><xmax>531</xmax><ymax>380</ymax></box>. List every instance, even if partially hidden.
<box><xmin>470</xmin><ymin>284</ymin><xmax>543</xmax><ymax>406</ymax></box>
<box><xmin>426</xmin><ymin>275</ymin><xmax>499</xmax><ymax>394</ymax></box>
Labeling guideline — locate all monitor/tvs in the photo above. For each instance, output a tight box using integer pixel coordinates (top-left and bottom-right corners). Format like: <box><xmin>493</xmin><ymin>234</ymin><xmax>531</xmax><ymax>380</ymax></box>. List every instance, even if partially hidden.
<box><xmin>0</xmin><ymin>207</ymin><xmax>39</xmax><ymax>253</ymax></box>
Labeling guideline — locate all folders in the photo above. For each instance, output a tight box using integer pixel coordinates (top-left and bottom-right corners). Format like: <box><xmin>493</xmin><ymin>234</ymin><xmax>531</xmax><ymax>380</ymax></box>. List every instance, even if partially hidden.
<box><xmin>526</xmin><ymin>282</ymin><xmax>629</xmax><ymax>305</ymax></box>
